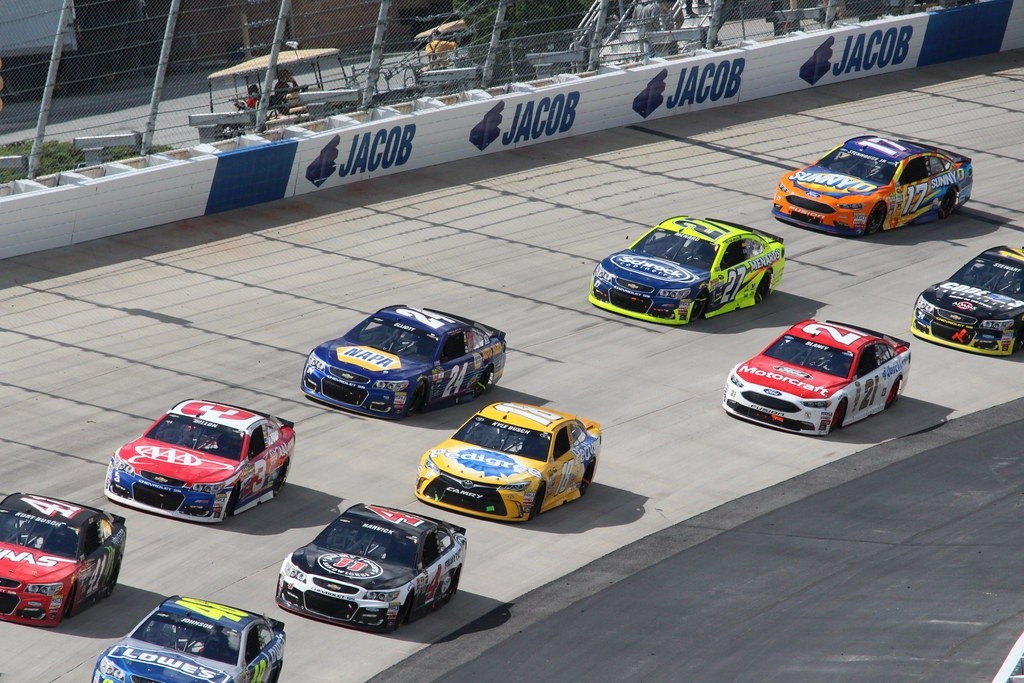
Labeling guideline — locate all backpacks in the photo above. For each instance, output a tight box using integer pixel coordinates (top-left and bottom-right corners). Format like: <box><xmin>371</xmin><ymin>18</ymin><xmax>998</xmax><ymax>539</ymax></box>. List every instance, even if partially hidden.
<box><xmin>428</xmin><ymin>40</ymin><xmax>442</xmax><ymax>68</ymax></box>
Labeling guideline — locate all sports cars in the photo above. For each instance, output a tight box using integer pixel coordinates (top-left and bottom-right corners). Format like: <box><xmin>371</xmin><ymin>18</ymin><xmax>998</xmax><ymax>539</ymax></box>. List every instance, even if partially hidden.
<box><xmin>415</xmin><ymin>399</ymin><xmax>602</xmax><ymax>524</ymax></box>
<box><xmin>909</xmin><ymin>245</ymin><xmax>1024</xmax><ymax>356</ymax></box>
<box><xmin>297</xmin><ymin>303</ymin><xmax>508</xmax><ymax>423</ymax></box>
<box><xmin>586</xmin><ymin>214</ymin><xmax>787</xmax><ymax>327</ymax></box>
<box><xmin>90</xmin><ymin>593</ymin><xmax>286</xmax><ymax>683</ymax></box>
<box><xmin>103</xmin><ymin>396</ymin><xmax>297</xmax><ymax>524</ymax></box>
<box><xmin>273</xmin><ymin>502</ymin><xmax>468</xmax><ymax>635</ymax></box>
<box><xmin>720</xmin><ymin>319</ymin><xmax>912</xmax><ymax>437</ymax></box>
<box><xmin>0</xmin><ymin>491</ymin><xmax>128</xmax><ymax>629</ymax></box>
<box><xmin>771</xmin><ymin>133</ymin><xmax>974</xmax><ymax>237</ymax></box>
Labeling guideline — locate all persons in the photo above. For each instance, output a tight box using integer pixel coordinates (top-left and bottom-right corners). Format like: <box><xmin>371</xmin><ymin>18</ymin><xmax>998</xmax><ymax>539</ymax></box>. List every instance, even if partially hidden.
<box><xmin>247</xmin><ymin>84</ymin><xmax>261</xmax><ymax>109</ymax></box>
<box><xmin>685</xmin><ymin>0</ymin><xmax>709</xmax><ymax>17</ymax></box>
<box><xmin>275</xmin><ymin>70</ymin><xmax>298</xmax><ymax>115</ymax></box>
<box><xmin>425</xmin><ymin>30</ymin><xmax>458</xmax><ymax>69</ymax></box>
<box><xmin>661</xmin><ymin>0</ymin><xmax>684</xmax><ymax>55</ymax></box>
<box><xmin>633</xmin><ymin>0</ymin><xmax>661</xmax><ymax>57</ymax></box>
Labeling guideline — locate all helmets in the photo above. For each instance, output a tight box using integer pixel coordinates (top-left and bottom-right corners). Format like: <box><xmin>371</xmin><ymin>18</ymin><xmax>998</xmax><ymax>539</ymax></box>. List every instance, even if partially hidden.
<box><xmin>393</xmin><ymin>530</ymin><xmax>416</xmax><ymax>555</ymax></box>
<box><xmin>227</xmin><ymin>428</ymin><xmax>242</xmax><ymax>447</ymax></box>
<box><xmin>54</xmin><ymin>529</ymin><xmax>77</xmax><ymax>551</ymax></box>
<box><xmin>204</xmin><ymin>633</ymin><xmax>228</xmax><ymax>653</ymax></box>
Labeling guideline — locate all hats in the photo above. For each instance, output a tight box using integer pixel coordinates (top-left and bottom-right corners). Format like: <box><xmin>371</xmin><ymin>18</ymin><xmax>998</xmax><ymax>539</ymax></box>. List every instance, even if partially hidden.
<box><xmin>433</xmin><ymin>30</ymin><xmax>441</xmax><ymax>35</ymax></box>
<box><xmin>277</xmin><ymin>69</ymin><xmax>294</xmax><ymax>79</ymax></box>
